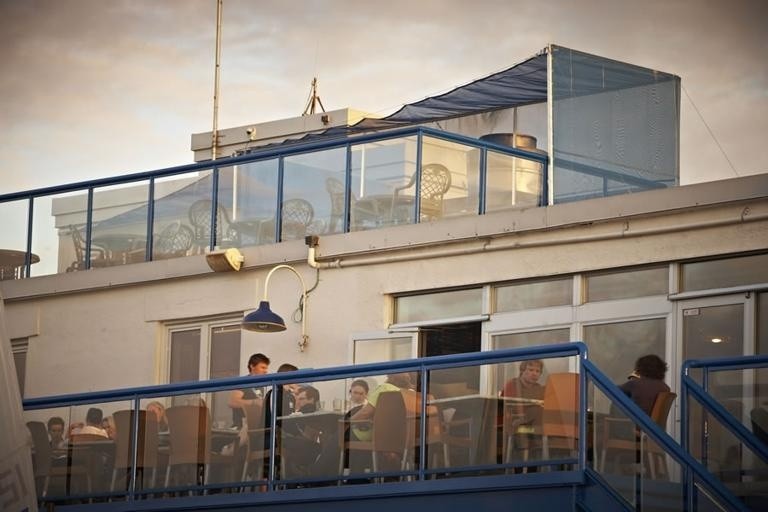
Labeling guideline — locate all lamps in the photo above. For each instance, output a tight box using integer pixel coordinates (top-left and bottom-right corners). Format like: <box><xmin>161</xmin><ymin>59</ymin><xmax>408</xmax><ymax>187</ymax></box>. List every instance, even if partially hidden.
<box><xmin>321</xmin><ymin>115</ymin><xmax>330</xmax><ymax>125</ymax></box>
<box><xmin>243</xmin><ymin>265</ymin><xmax>309</xmax><ymax>353</ymax></box>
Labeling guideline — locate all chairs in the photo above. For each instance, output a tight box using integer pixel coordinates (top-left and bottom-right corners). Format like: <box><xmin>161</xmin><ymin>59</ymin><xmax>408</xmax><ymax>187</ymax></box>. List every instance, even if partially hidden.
<box><xmin>66</xmin><ymin>163</ymin><xmax>451</xmax><ymax>272</ymax></box>
<box><xmin>25</xmin><ymin>372</ymin><xmax>677</xmax><ymax>503</ymax></box>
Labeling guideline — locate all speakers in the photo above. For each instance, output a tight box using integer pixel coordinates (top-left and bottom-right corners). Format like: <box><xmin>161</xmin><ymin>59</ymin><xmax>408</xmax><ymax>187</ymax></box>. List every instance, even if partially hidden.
<box><xmin>206</xmin><ymin>248</ymin><xmax>243</xmax><ymax>274</ymax></box>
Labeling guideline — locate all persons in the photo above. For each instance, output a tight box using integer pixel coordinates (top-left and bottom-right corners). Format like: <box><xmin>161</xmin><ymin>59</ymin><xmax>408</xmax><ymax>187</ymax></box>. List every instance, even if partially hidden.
<box><xmin>500</xmin><ymin>360</ymin><xmax>545</xmax><ymax>400</ymax></box>
<box><xmin>46</xmin><ymin>401</ymin><xmax>168</xmax><ymax>466</ymax></box>
<box><xmin>226</xmin><ymin>354</ymin><xmax>321</xmax><ymax>490</ymax></box>
<box><xmin>343</xmin><ymin>369</ymin><xmax>421</xmax><ymax>484</ymax></box>
<box><xmin>594</xmin><ymin>353</ymin><xmax>672</xmax><ymax>437</ymax></box>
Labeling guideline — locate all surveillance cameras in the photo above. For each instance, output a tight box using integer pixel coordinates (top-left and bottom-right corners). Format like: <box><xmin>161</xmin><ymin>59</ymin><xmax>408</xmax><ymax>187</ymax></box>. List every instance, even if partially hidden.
<box><xmin>245</xmin><ymin>126</ymin><xmax>255</xmax><ymax>137</ymax></box>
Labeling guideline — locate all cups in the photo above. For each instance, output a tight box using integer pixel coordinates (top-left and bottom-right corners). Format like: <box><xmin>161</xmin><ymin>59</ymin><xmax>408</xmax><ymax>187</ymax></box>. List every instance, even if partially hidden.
<box><xmin>333</xmin><ymin>398</ymin><xmax>342</xmax><ymax>411</ymax></box>
